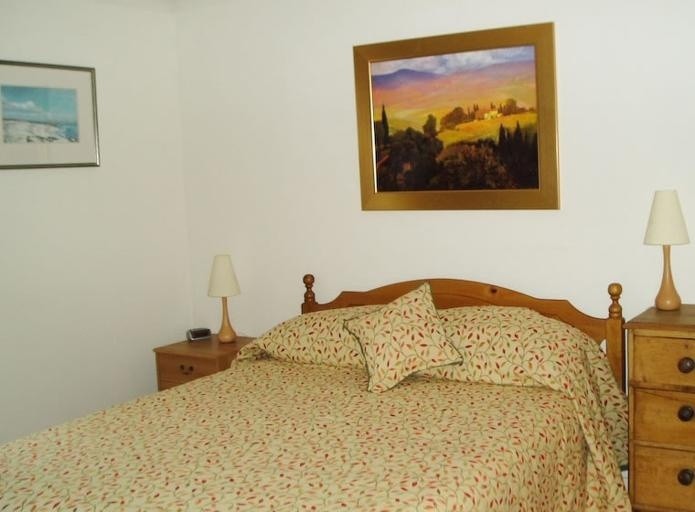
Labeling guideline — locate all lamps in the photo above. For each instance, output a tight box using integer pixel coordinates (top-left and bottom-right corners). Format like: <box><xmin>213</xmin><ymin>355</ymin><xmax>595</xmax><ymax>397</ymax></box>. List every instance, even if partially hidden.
<box><xmin>207</xmin><ymin>255</ymin><xmax>242</xmax><ymax>342</ymax></box>
<box><xmin>643</xmin><ymin>191</ymin><xmax>689</xmax><ymax>311</ymax></box>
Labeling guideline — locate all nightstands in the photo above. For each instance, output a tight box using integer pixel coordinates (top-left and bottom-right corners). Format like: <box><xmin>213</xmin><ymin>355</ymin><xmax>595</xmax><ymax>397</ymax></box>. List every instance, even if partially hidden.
<box><xmin>623</xmin><ymin>305</ymin><xmax>695</xmax><ymax>512</ymax></box>
<box><xmin>152</xmin><ymin>334</ymin><xmax>257</xmax><ymax>390</ymax></box>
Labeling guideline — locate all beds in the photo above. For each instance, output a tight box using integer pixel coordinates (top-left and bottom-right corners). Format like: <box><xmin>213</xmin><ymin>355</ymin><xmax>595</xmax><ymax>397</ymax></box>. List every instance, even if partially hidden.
<box><xmin>0</xmin><ymin>273</ymin><xmax>625</xmax><ymax>512</ymax></box>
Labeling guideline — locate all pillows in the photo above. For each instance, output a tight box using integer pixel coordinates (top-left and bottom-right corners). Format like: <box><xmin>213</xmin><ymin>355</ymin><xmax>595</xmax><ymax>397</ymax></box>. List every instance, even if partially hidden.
<box><xmin>343</xmin><ymin>281</ymin><xmax>463</xmax><ymax>393</ymax></box>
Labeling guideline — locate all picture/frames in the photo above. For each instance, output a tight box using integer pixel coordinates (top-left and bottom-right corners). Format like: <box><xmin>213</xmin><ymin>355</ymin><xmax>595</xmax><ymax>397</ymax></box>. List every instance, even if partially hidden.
<box><xmin>352</xmin><ymin>21</ymin><xmax>560</xmax><ymax>210</ymax></box>
<box><xmin>0</xmin><ymin>59</ymin><xmax>100</xmax><ymax>169</ymax></box>
